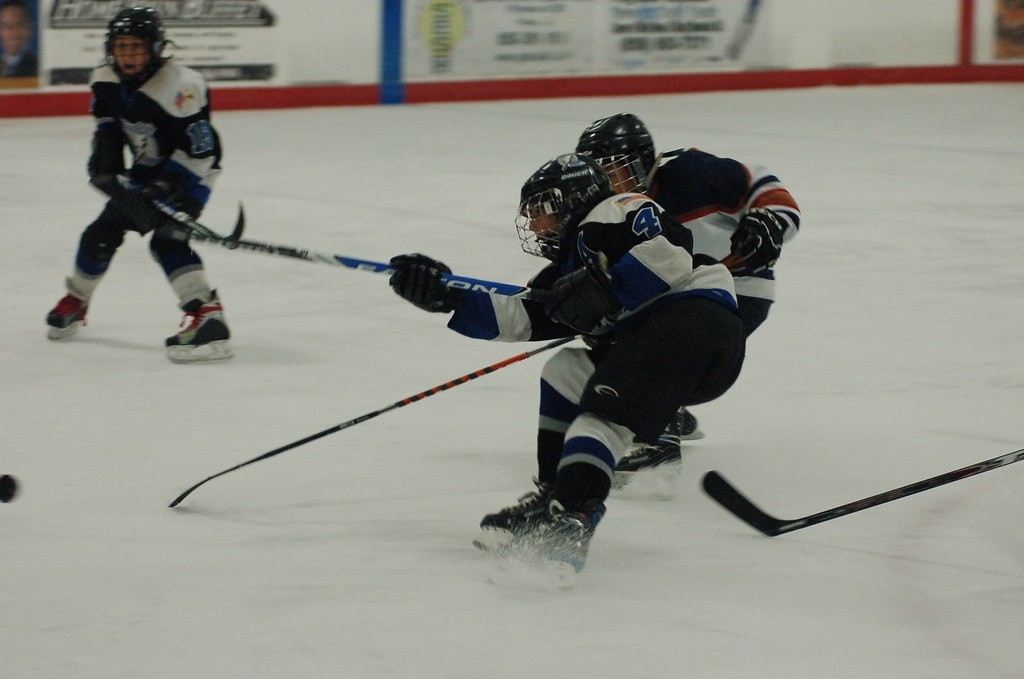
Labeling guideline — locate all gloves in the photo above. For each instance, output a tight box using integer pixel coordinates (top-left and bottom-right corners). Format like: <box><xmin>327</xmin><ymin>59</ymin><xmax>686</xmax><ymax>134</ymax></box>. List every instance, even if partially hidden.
<box><xmin>137</xmin><ymin>178</ymin><xmax>173</xmax><ymax>215</ymax></box>
<box><xmin>387</xmin><ymin>254</ymin><xmax>455</xmax><ymax>313</ymax></box>
<box><xmin>729</xmin><ymin>207</ymin><xmax>788</xmax><ymax>273</ymax></box>
<box><xmin>549</xmin><ymin>259</ymin><xmax>618</xmax><ymax>333</ymax></box>
<box><xmin>90</xmin><ymin>173</ymin><xmax>135</xmax><ymax>206</ymax></box>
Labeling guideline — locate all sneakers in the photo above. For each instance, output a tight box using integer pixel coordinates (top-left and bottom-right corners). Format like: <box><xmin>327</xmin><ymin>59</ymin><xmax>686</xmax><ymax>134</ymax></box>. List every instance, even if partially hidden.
<box><xmin>473</xmin><ymin>476</ymin><xmax>554</xmax><ymax>550</ymax></box>
<box><xmin>661</xmin><ymin>408</ymin><xmax>701</xmax><ymax>440</ymax></box>
<box><xmin>507</xmin><ymin>496</ymin><xmax>607</xmax><ymax>591</ymax></box>
<box><xmin>611</xmin><ymin>441</ymin><xmax>683</xmax><ymax>490</ymax></box>
<box><xmin>47</xmin><ymin>275</ymin><xmax>97</xmax><ymax>338</ymax></box>
<box><xmin>164</xmin><ymin>288</ymin><xmax>232</xmax><ymax>361</ymax></box>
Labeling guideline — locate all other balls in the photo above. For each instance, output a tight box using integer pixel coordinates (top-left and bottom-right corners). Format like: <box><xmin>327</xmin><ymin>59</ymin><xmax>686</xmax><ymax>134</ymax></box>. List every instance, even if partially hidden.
<box><xmin>0</xmin><ymin>474</ymin><xmax>17</xmax><ymax>503</ymax></box>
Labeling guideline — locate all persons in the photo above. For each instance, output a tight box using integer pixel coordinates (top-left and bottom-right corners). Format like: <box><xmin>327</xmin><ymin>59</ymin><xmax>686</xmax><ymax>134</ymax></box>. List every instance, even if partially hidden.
<box><xmin>0</xmin><ymin>0</ymin><xmax>38</xmax><ymax>78</ymax></box>
<box><xmin>47</xmin><ymin>4</ymin><xmax>236</xmax><ymax>364</ymax></box>
<box><xmin>575</xmin><ymin>112</ymin><xmax>802</xmax><ymax>489</ymax></box>
<box><xmin>388</xmin><ymin>153</ymin><xmax>746</xmax><ymax>584</ymax></box>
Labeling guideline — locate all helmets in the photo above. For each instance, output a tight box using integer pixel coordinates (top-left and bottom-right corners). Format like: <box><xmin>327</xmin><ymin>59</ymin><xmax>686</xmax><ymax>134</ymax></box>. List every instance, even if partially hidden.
<box><xmin>575</xmin><ymin>112</ymin><xmax>657</xmax><ymax>194</ymax></box>
<box><xmin>104</xmin><ymin>7</ymin><xmax>165</xmax><ymax>59</ymax></box>
<box><xmin>519</xmin><ymin>154</ymin><xmax>613</xmax><ymax>237</ymax></box>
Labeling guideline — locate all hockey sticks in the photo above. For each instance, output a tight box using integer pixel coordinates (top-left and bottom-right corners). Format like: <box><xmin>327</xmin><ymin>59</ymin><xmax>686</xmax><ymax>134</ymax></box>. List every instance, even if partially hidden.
<box><xmin>158</xmin><ymin>220</ymin><xmax>531</xmax><ymax>300</ymax></box>
<box><xmin>115</xmin><ymin>173</ymin><xmax>247</xmax><ymax>251</ymax></box>
<box><xmin>699</xmin><ymin>448</ymin><xmax>1024</xmax><ymax>538</ymax></box>
<box><xmin>169</xmin><ymin>331</ymin><xmax>581</xmax><ymax>508</ymax></box>
